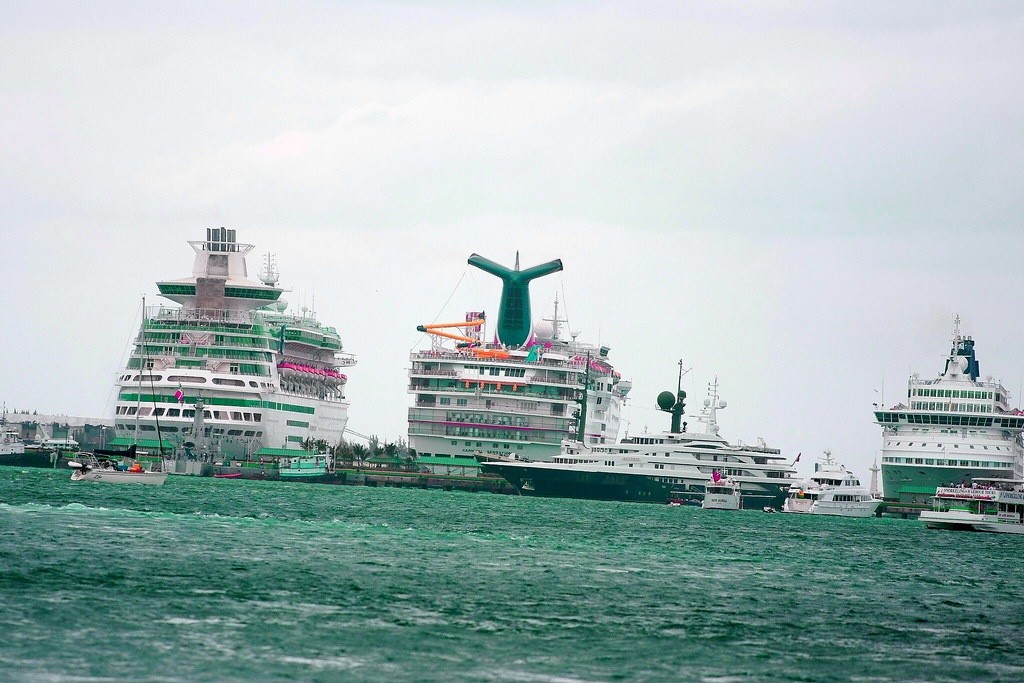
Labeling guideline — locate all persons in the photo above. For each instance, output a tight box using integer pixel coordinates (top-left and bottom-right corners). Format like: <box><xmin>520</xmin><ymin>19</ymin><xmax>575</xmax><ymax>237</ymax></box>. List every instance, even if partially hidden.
<box><xmin>941</xmin><ymin>479</ymin><xmax>1024</xmax><ymax>493</ymax></box>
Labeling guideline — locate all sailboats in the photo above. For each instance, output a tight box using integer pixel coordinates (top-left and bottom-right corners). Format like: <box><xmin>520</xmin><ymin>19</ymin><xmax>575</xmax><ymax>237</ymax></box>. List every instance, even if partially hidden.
<box><xmin>68</xmin><ymin>296</ymin><xmax>171</xmax><ymax>485</ymax></box>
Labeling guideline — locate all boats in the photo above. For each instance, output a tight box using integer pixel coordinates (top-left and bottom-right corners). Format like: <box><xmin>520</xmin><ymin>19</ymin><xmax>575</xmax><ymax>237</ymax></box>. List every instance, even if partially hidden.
<box><xmin>277</xmin><ymin>454</ymin><xmax>347</xmax><ymax>485</ymax></box>
<box><xmin>0</xmin><ymin>417</ymin><xmax>23</xmax><ymax>454</ymax></box>
<box><xmin>702</xmin><ymin>471</ymin><xmax>741</xmax><ymax>510</ymax></box>
<box><xmin>918</xmin><ymin>478</ymin><xmax>1024</xmax><ymax>534</ymax></box>
<box><xmin>407</xmin><ymin>250</ymin><xmax>634</xmax><ymax>476</ymax></box>
<box><xmin>215</xmin><ymin>472</ymin><xmax>243</xmax><ymax>478</ymax></box>
<box><xmin>666</xmin><ymin>491</ymin><xmax>705</xmax><ymax>506</ymax></box>
<box><xmin>277</xmin><ymin>360</ymin><xmax>347</xmax><ymax>386</ymax></box>
<box><xmin>474</xmin><ymin>360</ymin><xmax>804</xmax><ymax>512</ymax></box>
<box><xmin>781</xmin><ymin>449</ymin><xmax>883</xmax><ymax>518</ymax></box>
<box><xmin>873</xmin><ymin>314</ymin><xmax>1024</xmax><ymax>514</ymax></box>
<box><xmin>570</xmin><ymin>356</ymin><xmax>621</xmax><ymax>385</ymax></box>
<box><xmin>25</xmin><ymin>440</ymin><xmax>79</xmax><ymax>450</ymax></box>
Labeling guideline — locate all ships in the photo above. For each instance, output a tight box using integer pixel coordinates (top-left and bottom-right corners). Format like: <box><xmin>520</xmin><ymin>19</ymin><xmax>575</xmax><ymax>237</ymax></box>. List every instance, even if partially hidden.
<box><xmin>114</xmin><ymin>228</ymin><xmax>358</xmax><ymax>461</ymax></box>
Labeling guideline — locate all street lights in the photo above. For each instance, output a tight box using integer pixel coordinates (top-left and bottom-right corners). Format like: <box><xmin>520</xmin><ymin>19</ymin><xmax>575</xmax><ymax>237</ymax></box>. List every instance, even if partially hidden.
<box><xmin>102</xmin><ymin>427</ymin><xmax>107</xmax><ymax>449</ymax></box>
